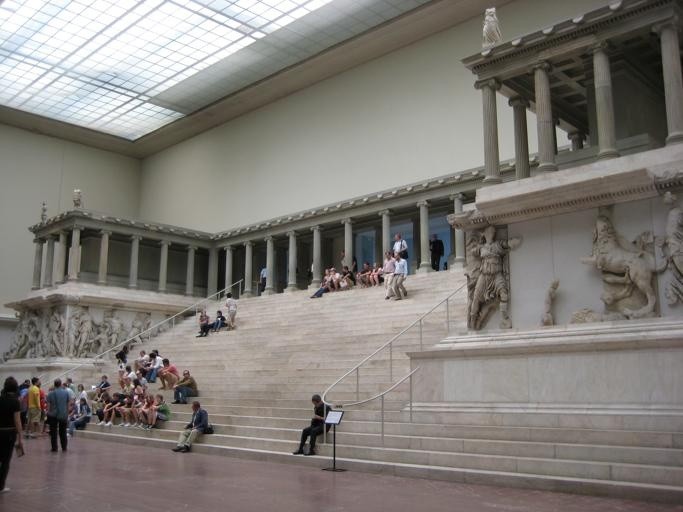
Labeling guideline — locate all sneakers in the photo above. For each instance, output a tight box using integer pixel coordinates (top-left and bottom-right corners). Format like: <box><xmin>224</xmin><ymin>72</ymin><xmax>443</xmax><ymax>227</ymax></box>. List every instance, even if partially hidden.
<box><xmin>171</xmin><ymin>400</ymin><xmax>179</xmax><ymax>404</ymax></box>
<box><xmin>66</xmin><ymin>428</ymin><xmax>76</xmax><ymax>433</ymax></box>
<box><xmin>22</xmin><ymin>430</ymin><xmax>49</xmax><ymax>439</ymax></box>
<box><xmin>179</xmin><ymin>400</ymin><xmax>186</xmax><ymax>404</ymax></box>
<box><xmin>360</xmin><ymin>283</ymin><xmax>379</xmax><ymax>289</ymax></box>
<box><xmin>66</xmin><ymin>432</ymin><xmax>73</xmax><ymax>437</ymax></box>
<box><xmin>96</xmin><ymin>421</ymin><xmax>156</xmax><ymax>431</ymax></box>
<box><xmin>309</xmin><ymin>295</ymin><xmax>317</xmax><ymax>299</ymax></box>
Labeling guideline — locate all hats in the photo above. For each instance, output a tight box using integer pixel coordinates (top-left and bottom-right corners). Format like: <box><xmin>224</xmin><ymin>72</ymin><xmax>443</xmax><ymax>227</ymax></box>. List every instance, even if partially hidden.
<box><xmin>328</xmin><ymin>268</ymin><xmax>335</xmax><ymax>272</ymax></box>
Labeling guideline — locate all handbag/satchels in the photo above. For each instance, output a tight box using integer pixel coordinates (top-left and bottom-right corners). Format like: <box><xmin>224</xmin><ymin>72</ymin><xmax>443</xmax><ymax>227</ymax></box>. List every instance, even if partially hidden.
<box><xmin>203</xmin><ymin>425</ymin><xmax>214</xmax><ymax>434</ymax></box>
<box><xmin>46</xmin><ymin>411</ymin><xmax>57</xmax><ymax>425</ymax></box>
<box><xmin>399</xmin><ymin>250</ymin><xmax>408</xmax><ymax>259</ymax></box>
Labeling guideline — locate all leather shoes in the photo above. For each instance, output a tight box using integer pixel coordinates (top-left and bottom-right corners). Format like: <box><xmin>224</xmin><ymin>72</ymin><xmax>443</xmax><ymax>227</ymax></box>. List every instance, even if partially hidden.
<box><xmin>62</xmin><ymin>448</ymin><xmax>67</xmax><ymax>451</ymax></box>
<box><xmin>384</xmin><ymin>292</ymin><xmax>406</xmax><ymax>301</ymax></box>
<box><xmin>180</xmin><ymin>447</ymin><xmax>190</xmax><ymax>453</ymax></box>
<box><xmin>171</xmin><ymin>447</ymin><xmax>182</xmax><ymax>452</ymax></box>
<box><xmin>1</xmin><ymin>487</ymin><xmax>10</xmax><ymax>493</ymax></box>
<box><xmin>292</xmin><ymin>450</ymin><xmax>302</xmax><ymax>455</ymax></box>
<box><xmin>303</xmin><ymin>451</ymin><xmax>313</xmax><ymax>456</ymax></box>
<box><xmin>50</xmin><ymin>449</ymin><xmax>58</xmax><ymax>452</ymax></box>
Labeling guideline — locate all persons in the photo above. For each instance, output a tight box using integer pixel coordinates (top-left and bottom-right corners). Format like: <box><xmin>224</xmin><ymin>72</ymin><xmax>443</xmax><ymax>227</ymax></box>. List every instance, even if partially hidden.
<box><xmin>309</xmin><ymin>233</ymin><xmax>409</xmax><ymax>300</ymax></box>
<box><xmin>195</xmin><ymin>293</ymin><xmax>237</xmax><ymax>337</ymax></box>
<box><xmin>0</xmin><ymin>377</ymin><xmax>24</xmax><ymax>493</ymax></box>
<box><xmin>18</xmin><ymin>345</ymin><xmax>199</xmax><ymax>453</ymax></box>
<box><xmin>655</xmin><ymin>191</ymin><xmax>683</xmax><ymax>306</ymax></box>
<box><xmin>470</xmin><ymin>225</ymin><xmax>523</xmax><ymax>330</ymax></box>
<box><xmin>430</xmin><ymin>233</ymin><xmax>444</xmax><ymax>271</ymax></box>
<box><xmin>293</xmin><ymin>394</ymin><xmax>332</xmax><ymax>455</ymax></box>
<box><xmin>171</xmin><ymin>401</ymin><xmax>208</xmax><ymax>453</ymax></box>
<box><xmin>259</xmin><ymin>268</ymin><xmax>266</xmax><ymax>295</ymax></box>
